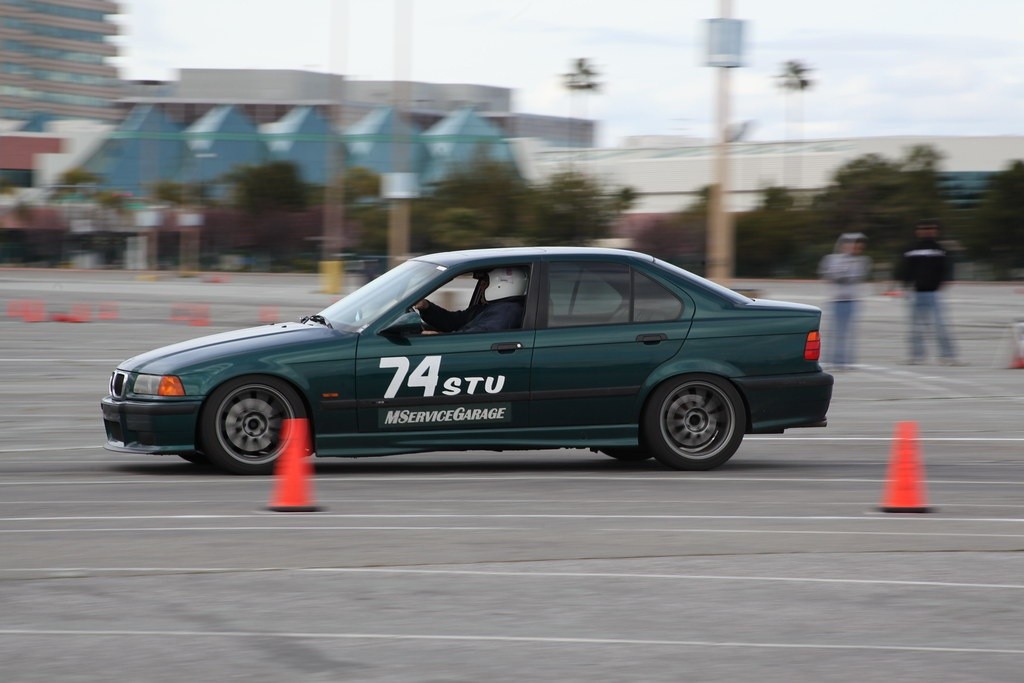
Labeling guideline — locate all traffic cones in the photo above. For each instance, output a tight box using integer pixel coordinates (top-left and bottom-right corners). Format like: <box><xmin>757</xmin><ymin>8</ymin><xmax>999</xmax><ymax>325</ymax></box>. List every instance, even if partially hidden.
<box><xmin>261</xmin><ymin>419</ymin><xmax>328</xmax><ymax>512</ymax></box>
<box><xmin>872</xmin><ymin>421</ymin><xmax>934</xmax><ymax>513</ymax></box>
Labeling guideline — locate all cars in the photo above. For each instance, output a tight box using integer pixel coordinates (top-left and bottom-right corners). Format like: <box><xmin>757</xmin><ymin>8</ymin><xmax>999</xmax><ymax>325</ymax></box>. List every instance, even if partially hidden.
<box><xmin>98</xmin><ymin>247</ymin><xmax>836</xmax><ymax>485</ymax></box>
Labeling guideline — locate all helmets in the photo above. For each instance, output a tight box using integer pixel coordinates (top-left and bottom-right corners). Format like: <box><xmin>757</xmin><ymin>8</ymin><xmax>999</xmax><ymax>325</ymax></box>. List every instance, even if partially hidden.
<box><xmin>484</xmin><ymin>268</ymin><xmax>529</xmax><ymax>302</ymax></box>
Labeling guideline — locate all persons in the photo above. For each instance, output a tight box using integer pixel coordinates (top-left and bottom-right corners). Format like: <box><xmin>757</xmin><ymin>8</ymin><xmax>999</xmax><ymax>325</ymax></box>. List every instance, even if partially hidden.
<box><xmin>414</xmin><ymin>266</ymin><xmax>528</xmax><ymax>335</ymax></box>
<box><xmin>894</xmin><ymin>218</ymin><xmax>965</xmax><ymax>366</ymax></box>
<box><xmin>820</xmin><ymin>233</ymin><xmax>874</xmax><ymax>365</ymax></box>
